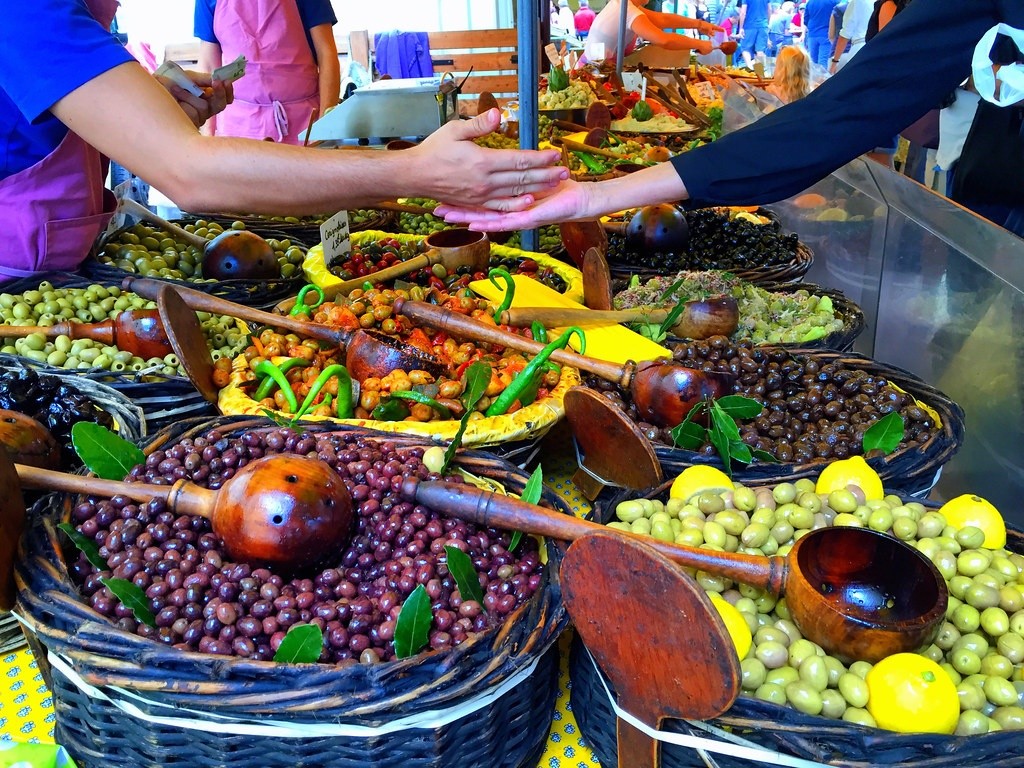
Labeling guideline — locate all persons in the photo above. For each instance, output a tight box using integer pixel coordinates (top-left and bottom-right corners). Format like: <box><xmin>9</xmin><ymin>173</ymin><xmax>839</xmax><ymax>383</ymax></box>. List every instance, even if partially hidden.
<box><xmin>0</xmin><ymin>0</ymin><xmax>574</xmax><ymax>291</ymax></box>
<box><xmin>547</xmin><ymin>1</ymin><xmax>945</xmax><ymax>190</ymax></box>
<box><xmin>191</xmin><ymin>0</ymin><xmax>342</xmax><ymax>148</ymax></box>
<box><xmin>433</xmin><ymin>0</ymin><xmax>1024</xmax><ymax>381</ymax></box>
